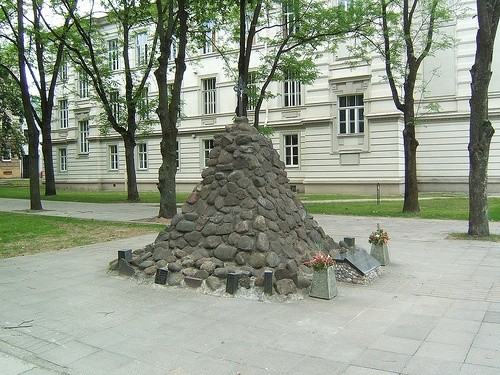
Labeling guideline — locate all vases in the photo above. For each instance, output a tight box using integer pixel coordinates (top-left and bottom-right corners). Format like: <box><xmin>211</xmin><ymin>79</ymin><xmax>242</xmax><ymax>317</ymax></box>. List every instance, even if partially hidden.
<box><xmin>308</xmin><ymin>266</ymin><xmax>339</xmax><ymax>300</ymax></box>
<box><xmin>369</xmin><ymin>243</ymin><xmax>391</xmax><ymax>266</ymax></box>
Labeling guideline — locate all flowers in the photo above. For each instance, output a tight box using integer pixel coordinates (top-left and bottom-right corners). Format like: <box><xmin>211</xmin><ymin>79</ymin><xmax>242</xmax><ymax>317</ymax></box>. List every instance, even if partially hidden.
<box><xmin>369</xmin><ymin>222</ymin><xmax>391</xmax><ymax>245</ymax></box>
<box><xmin>306</xmin><ymin>248</ymin><xmax>335</xmax><ymax>268</ymax></box>
